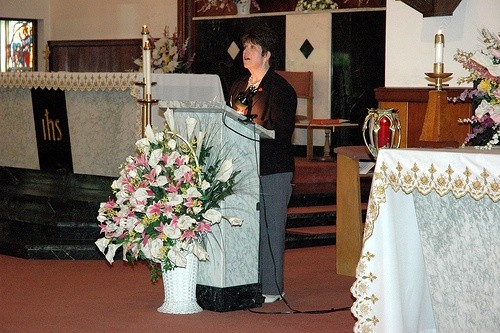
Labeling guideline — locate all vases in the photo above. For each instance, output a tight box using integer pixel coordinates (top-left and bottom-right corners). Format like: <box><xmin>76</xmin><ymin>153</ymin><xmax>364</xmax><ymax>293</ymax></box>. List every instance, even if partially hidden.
<box><xmin>363</xmin><ymin>108</ymin><xmax>401</xmax><ymax>161</ymax></box>
<box><xmin>236</xmin><ymin>0</ymin><xmax>251</xmax><ymax>16</ymax></box>
<box><xmin>157</xmin><ymin>254</ymin><xmax>203</xmax><ymax>314</ymax></box>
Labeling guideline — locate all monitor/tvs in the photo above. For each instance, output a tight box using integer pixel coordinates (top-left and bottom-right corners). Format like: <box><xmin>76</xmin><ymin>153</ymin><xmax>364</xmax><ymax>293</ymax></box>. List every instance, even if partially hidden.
<box><xmin>0</xmin><ymin>17</ymin><xmax>38</xmax><ymax>72</ymax></box>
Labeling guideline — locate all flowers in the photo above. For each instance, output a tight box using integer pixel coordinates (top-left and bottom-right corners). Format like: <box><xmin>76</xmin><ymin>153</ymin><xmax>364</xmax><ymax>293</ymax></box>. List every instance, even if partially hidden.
<box><xmin>295</xmin><ymin>0</ymin><xmax>338</xmax><ymax>11</ymax></box>
<box><xmin>95</xmin><ymin>107</ymin><xmax>244</xmax><ymax>285</ymax></box>
<box><xmin>134</xmin><ymin>25</ymin><xmax>196</xmax><ymax>73</ymax></box>
<box><xmin>198</xmin><ymin>0</ymin><xmax>260</xmax><ymax>16</ymax></box>
<box><xmin>447</xmin><ymin>27</ymin><xmax>500</xmax><ymax>150</ymax></box>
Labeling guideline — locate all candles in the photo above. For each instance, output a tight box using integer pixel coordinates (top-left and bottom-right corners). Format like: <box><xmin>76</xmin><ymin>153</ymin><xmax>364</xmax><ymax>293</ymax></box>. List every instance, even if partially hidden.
<box><xmin>137</xmin><ymin>41</ymin><xmax>161</xmax><ymax>103</ymax></box>
<box><xmin>135</xmin><ymin>24</ymin><xmax>158</xmax><ymax>85</ymax></box>
<box><xmin>423</xmin><ymin>29</ymin><xmax>453</xmax><ymax>91</ymax></box>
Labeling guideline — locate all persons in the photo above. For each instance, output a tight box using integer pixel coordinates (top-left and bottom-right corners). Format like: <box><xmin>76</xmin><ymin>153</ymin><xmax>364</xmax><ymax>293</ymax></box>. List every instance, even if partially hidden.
<box><xmin>227</xmin><ymin>31</ymin><xmax>297</xmax><ymax>303</ymax></box>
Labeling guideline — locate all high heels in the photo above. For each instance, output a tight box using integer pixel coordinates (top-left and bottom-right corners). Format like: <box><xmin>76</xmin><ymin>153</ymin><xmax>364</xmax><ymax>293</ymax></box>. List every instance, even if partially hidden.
<box><xmin>262</xmin><ymin>292</ymin><xmax>285</xmax><ymax>303</ymax></box>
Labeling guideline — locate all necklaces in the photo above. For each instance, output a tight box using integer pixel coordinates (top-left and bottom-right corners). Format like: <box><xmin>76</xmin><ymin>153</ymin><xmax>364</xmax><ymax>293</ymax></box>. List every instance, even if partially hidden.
<box><xmin>248</xmin><ymin>66</ymin><xmax>270</xmax><ymax>87</ymax></box>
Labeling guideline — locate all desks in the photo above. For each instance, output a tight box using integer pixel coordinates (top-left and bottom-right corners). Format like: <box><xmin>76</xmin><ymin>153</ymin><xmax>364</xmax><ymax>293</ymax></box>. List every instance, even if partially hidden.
<box><xmin>335</xmin><ymin>146</ymin><xmax>378</xmax><ymax>278</ymax></box>
<box><xmin>0</xmin><ymin>71</ymin><xmax>226</xmax><ymax>179</ymax></box>
<box><xmin>350</xmin><ymin>146</ymin><xmax>500</xmax><ymax>333</ymax></box>
